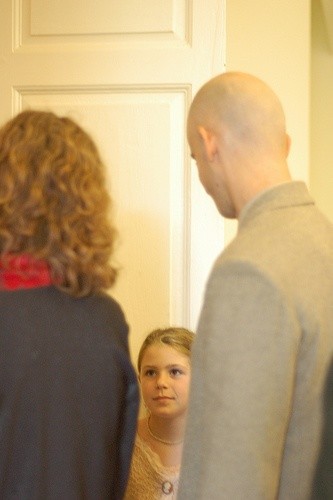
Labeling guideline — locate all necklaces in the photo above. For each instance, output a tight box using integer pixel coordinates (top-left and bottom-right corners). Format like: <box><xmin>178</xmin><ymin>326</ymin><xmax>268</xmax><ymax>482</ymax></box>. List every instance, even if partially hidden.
<box><xmin>146</xmin><ymin>415</ymin><xmax>182</xmax><ymax>445</ymax></box>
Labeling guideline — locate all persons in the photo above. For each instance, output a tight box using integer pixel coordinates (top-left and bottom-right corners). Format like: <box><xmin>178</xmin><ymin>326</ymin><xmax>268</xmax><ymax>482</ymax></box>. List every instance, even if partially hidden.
<box><xmin>124</xmin><ymin>328</ymin><xmax>196</xmax><ymax>500</ymax></box>
<box><xmin>0</xmin><ymin>109</ymin><xmax>140</xmax><ymax>500</ymax></box>
<box><xmin>178</xmin><ymin>72</ymin><xmax>333</xmax><ymax>500</ymax></box>
